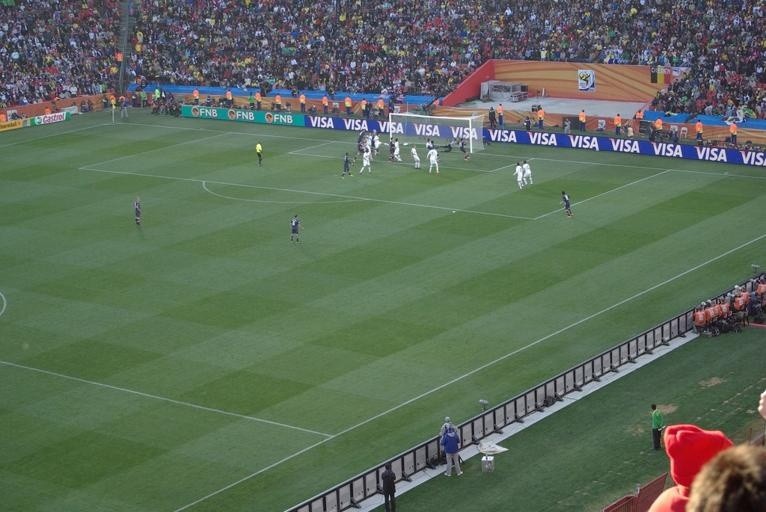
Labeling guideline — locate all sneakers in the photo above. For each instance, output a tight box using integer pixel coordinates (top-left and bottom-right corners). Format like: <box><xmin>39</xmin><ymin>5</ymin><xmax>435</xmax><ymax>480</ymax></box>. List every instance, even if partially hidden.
<box><xmin>457</xmin><ymin>471</ymin><xmax>463</xmax><ymax>476</ymax></box>
<box><xmin>444</xmin><ymin>473</ymin><xmax>452</xmax><ymax>477</ymax></box>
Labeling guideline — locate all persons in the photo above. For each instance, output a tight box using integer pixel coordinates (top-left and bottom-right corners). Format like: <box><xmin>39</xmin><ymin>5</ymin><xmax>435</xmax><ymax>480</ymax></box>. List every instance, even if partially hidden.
<box><xmin>425</xmin><ymin>147</ymin><xmax>440</xmax><ymax>175</ymax></box>
<box><xmin>691</xmin><ymin>272</ymin><xmax>765</xmax><ymax>332</ymax></box>
<box><xmin>686</xmin><ymin>442</ymin><xmax>766</xmax><ymax>512</ymax></box>
<box><xmin>360</xmin><ymin>147</ymin><xmax>371</xmax><ymax>175</ymax></box>
<box><xmin>135</xmin><ymin>197</ymin><xmax>143</xmax><ymax>224</ymax></box>
<box><xmin>752</xmin><ymin>391</ymin><xmax>765</xmax><ymax>443</ymax></box>
<box><xmin>291</xmin><ymin>213</ymin><xmax>301</xmax><ymax>242</ymax></box>
<box><xmin>513</xmin><ymin>164</ymin><xmax>524</xmax><ymax>188</ymax></box>
<box><xmin>437</xmin><ymin>415</ymin><xmax>456</xmax><ymax>443</ymax></box>
<box><xmin>522</xmin><ymin>161</ymin><xmax>533</xmax><ymax>186</ymax></box>
<box><xmin>340</xmin><ymin>154</ymin><xmax>355</xmax><ymax>176</ymax></box>
<box><xmin>0</xmin><ymin>0</ymin><xmax>766</xmax><ymax>161</ymax></box>
<box><xmin>647</xmin><ymin>425</ymin><xmax>734</xmax><ymax>511</ymax></box>
<box><xmin>650</xmin><ymin>403</ymin><xmax>665</xmax><ymax>448</ymax></box>
<box><xmin>440</xmin><ymin>423</ymin><xmax>464</xmax><ymax>477</ymax></box>
<box><xmin>382</xmin><ymin>460</ymin><xmax>396</xmax><ymax>510</ymax></box>
<box><xmin>410</xmin><ymin>146</ymin><xmax>420</xmax><ymax>169</ymax></box>
<box><xmin>559</xmin><ymin>190</ymin><xmax>573</xmax><ymax>215</ymax></box>
<box><xmin>255</xmin><ymin>142</ymin><xmax>264</xmax><ymax>164</ymax></box>
